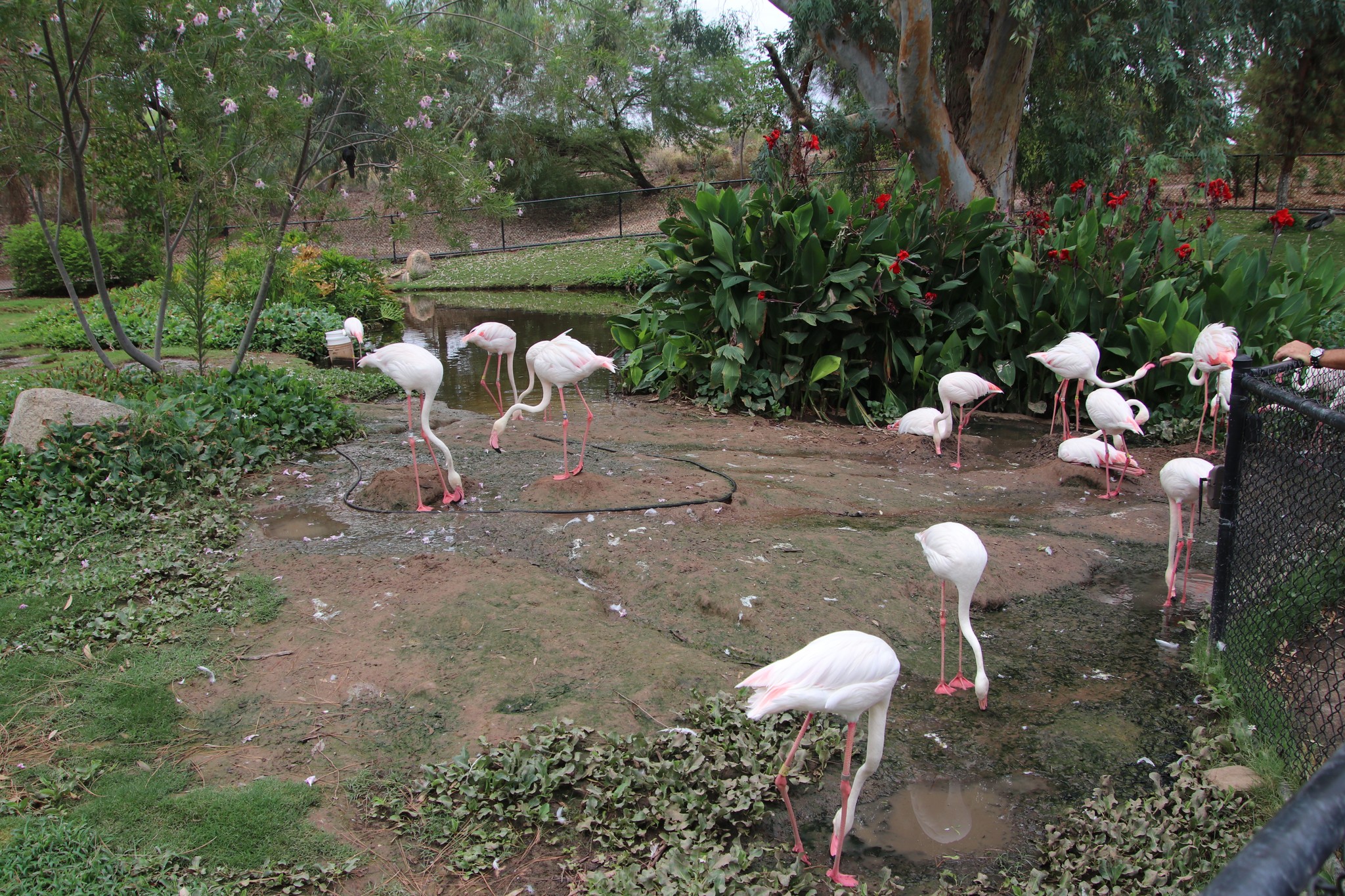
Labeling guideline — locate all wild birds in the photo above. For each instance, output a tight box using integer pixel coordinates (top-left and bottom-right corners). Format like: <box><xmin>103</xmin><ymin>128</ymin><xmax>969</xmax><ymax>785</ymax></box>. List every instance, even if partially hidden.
<box><xmin>341</xmin><ymin>316</ymin><xmax>364</xmax><ymax>361</ymax></box>
<box><xmin>888</xmin><ymin>397</ymin><xmax>953</xmax><ymax>441</ymax></box>
<box><xmin>932</xmin><ymin>369</ymin><xmax>1004</xmax><ymax>469</ymax></box>
<box><xmin>489</xmin><ymin>326</ymin><xmax>621</xmax><ymax>484</ymax></box>
<box><xmin>510</xmin><ymin>337</ymin><xmax>554</xmax><ymax>424</ymax></box>
<box><xmin>356</xmin><ymin>342</ymin><xmax>464</xmax><ymax>514</ymax></box>
<box><xmin>910</xmin><ymin>520</ymin><xmax>993</xmax><ymax>712</ymax></box>
<box><xmin>732</xmin><ymin>625</ymin><xmax>907</xmax><ymax>895</ymax></box>
<box><xmin>1025</xmin><ymin>317</ymin><xmax>1344</xmax><ymax>612</ymax></box>
<box><xmin>459</xmin><ymin>321</ymin><xmax>527</xmax><ymax>423</ymax></box>
